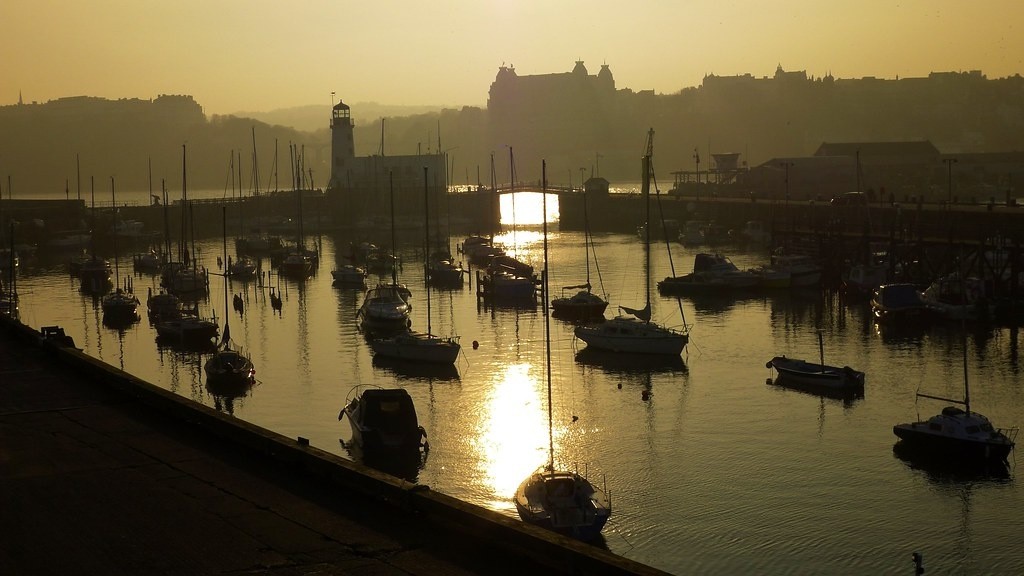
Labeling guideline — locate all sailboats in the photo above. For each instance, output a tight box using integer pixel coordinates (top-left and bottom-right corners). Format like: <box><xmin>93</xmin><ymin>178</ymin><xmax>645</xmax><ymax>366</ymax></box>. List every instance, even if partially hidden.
<box><xmin>891</xmin><ymin>224</ymin><xmax>1019</xmax><ymax>466</ymax></box>
<box><xmin>1</xmin><ymin>116</ymin><xmax>997</xmax><ymax>545</ymax></box>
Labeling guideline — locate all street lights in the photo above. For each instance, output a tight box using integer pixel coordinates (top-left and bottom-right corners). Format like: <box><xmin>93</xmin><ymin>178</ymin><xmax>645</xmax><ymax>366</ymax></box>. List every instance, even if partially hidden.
<box><xmin>780</xmin><ymin>160</ymin><xmax>795</xmax><ymax>208</ymax></box>
<box><xmin>579</xmin><ymin>167</ymin><xmax>587</xmax><ymax>191</ymax></box>
<box><xmin>942</xmin><ymin>158</ymin><xmax>957</xmax><ymax>211</ymax></box>
